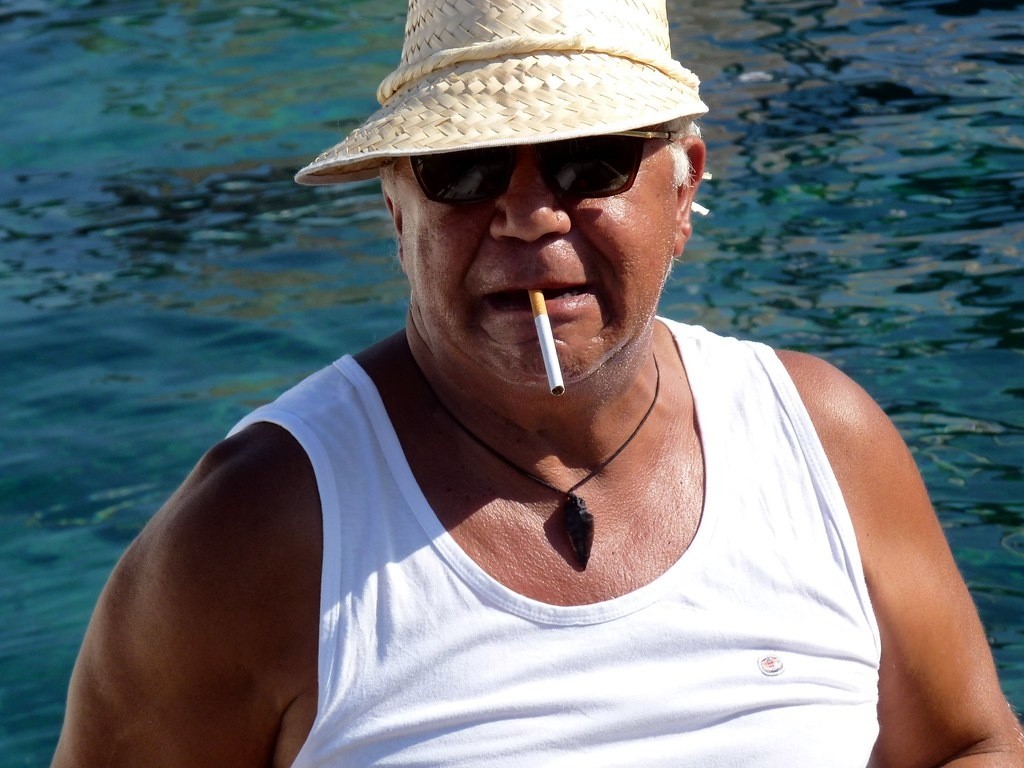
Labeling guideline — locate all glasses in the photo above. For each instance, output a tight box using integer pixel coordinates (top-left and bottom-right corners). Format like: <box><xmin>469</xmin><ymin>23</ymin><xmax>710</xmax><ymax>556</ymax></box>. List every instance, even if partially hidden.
<box><xmin>410</xmin><ymin>130</ymin><xmax>682</xmax><ymax>205</ymax></box>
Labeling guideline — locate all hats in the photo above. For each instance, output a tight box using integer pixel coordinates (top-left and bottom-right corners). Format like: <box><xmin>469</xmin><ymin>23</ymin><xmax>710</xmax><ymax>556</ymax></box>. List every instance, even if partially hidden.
<box><xmin>294</xmin><ymin>0</ymin><xmax>709</xmax><ymax>186</ymax></box>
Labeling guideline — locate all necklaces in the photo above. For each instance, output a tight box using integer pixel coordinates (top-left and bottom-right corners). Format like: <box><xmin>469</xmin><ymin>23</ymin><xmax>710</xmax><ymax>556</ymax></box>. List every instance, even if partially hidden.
<box><xmin>408</xmin><ymin>350</ymin><xmax>662</xmax><ymax>572</ymax></box>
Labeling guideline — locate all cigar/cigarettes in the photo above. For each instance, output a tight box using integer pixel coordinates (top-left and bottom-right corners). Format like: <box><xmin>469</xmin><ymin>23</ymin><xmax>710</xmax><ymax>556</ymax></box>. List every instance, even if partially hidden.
<box><xmin>526</xmin><ymin>288</ymin><xmax>566</xmax><ymax>397</ymax></box>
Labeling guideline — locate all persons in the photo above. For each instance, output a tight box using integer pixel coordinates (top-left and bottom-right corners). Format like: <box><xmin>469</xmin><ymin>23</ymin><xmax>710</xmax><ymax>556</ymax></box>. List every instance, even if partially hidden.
<box><xmin>48</xmin><ymin>0</ymin><xmax>1024</xmax><ymax>768</ymax></box>
